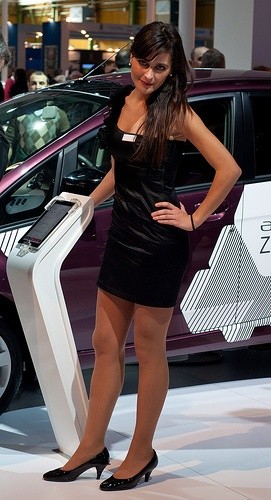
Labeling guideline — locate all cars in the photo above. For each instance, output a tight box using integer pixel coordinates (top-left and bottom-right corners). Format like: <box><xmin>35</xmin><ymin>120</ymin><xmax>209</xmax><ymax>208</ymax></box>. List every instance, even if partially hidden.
<box><xmin>2</xmin><ymin>43</ymin><xmax>270</xmax><ymax>410</ymax></box>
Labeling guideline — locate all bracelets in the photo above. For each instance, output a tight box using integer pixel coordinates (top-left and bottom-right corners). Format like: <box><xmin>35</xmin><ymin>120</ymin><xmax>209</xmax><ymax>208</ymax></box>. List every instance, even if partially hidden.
<box><xmin>191</xmin><ymin>214</ymin><xmax>196</xmax><ymax>231</ymax></box>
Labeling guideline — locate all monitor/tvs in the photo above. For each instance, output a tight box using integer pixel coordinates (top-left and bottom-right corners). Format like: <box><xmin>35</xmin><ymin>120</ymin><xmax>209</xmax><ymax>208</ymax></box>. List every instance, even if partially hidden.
<box><xmin>17</xmin><ymin>200</ymin><xmax>76</xmax><ymax>249</ymax></box>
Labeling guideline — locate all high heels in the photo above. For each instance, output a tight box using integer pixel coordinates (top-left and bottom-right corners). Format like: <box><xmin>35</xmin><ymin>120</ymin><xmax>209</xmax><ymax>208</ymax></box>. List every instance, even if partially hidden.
<box><xmin>42</xmin><ymin>446</ymin><xmax>110</xmax><ymax>482</ymax></box>
<box><xmin>99</xmin><ymin>448</ymin><xmax>159</xmax><ymax>492</ymax></box>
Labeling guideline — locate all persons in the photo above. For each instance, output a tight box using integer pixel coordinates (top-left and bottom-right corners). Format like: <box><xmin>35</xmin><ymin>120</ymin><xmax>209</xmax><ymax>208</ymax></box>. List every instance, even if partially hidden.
<box><xmin>0</xmin><ymin>31</ymin><xmax>271</xmax><ymax>181</ymax></box>
<box><xmin>42</xmin><ymin>21</ymin><xmax>242</xmax><ymax>492</ymax></box>
